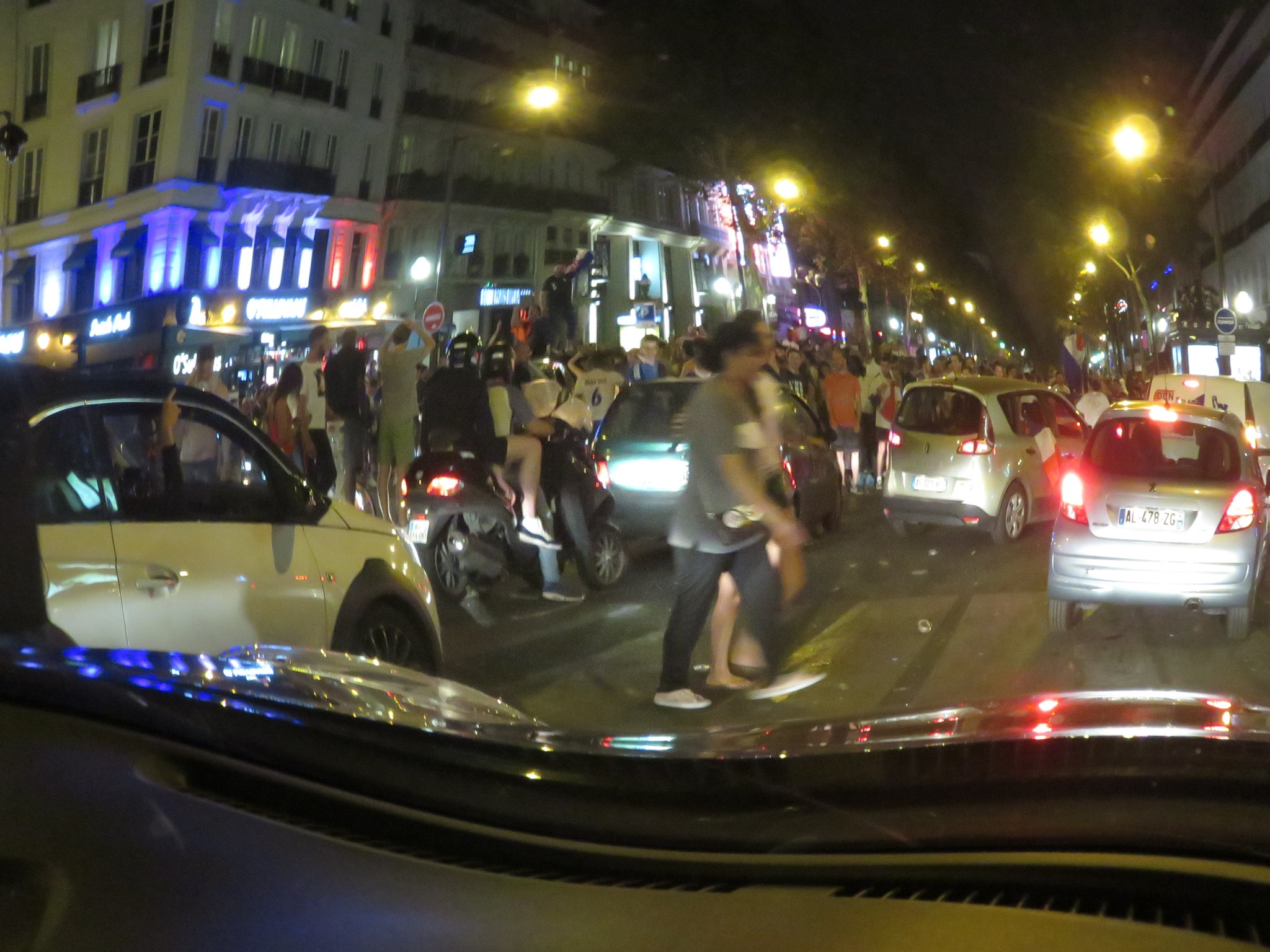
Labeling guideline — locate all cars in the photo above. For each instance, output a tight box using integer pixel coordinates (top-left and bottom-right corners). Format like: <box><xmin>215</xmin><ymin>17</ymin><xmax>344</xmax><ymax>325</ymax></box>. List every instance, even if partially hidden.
<box><xmin>590</xmin><ymin>377</ymin><xmax>846</xmax><ymax>560</ymax></box>
<box><xmin>4</xmin><ymin>360</ymin><xmax>445</xmax><ymax>672</ymax></box>
<box><xmin>881</xmin><ymin>377</ymin><xmax>1082</xmax><ymax>546</ymax></box>
<box><xmin>1045</xmin><ymin>399</ymin><xmax>1270</xmax><ymax>641</ymax></box>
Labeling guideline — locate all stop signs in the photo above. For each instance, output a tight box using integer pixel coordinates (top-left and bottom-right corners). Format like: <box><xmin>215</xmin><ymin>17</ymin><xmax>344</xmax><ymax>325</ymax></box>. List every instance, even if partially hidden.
<box><xmin>421</xmin><ymin>302</ymin><xmax>445</xmax><ymax>334</ymax></box>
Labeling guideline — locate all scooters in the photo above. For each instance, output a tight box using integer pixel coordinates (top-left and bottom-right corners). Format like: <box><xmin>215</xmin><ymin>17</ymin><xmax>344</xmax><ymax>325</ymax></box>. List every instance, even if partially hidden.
<box><xmin>401</xmin><ymin>387</ymin><xmax>629</xmax><ymax>605</ymax></box>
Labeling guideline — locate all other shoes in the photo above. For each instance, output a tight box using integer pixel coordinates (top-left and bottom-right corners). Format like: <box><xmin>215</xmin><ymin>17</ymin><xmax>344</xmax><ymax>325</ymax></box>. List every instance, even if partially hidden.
<box><xmin>519</xmin><ymin>526</ymin><xmax>563</xmax><ymax>549</ymax></box>
<box><xmin>543</xmin><ymin>582</ymin><xmax>585</xmax><ymax>602</ymax></box>
<box><xmin>654</xmin><ymin>688</ymin><xmax>711</xmax><ymax>709</ymax></box>
<box><xmin>462</xmin><ymin>594</ymin><xmax>489</xmax><ymax>625</ymax></box>
<box><xmin>756</xmin><ymin>673</ymin><xmax>826</xmax><ymax>700</ymax></box>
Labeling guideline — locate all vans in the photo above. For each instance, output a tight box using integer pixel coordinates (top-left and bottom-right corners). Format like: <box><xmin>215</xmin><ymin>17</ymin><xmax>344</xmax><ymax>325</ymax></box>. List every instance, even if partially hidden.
<box><xmin>1146</xmin><ymin>373</ymin><xmax>1270</xmax><ymax>496</ymax></box>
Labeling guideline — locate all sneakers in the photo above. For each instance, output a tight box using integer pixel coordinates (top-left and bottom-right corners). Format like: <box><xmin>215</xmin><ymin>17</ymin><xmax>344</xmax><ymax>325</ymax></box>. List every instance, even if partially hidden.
<box><xmin>849</xmin><ymin>486</ymin><xmax>865</xmax><ymax>495</ymax></box>
<box><xmin>876</xmin><ymin>480</ymin><xmax>882</xmax><ymax>490</ymax></box>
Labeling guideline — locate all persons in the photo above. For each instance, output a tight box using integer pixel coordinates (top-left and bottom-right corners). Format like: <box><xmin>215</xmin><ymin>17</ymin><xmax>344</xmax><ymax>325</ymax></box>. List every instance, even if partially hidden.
<box><xmin>100</xmin><ymin>260</ymin><xmax>1160</xmax><ymax>710</ymax></box>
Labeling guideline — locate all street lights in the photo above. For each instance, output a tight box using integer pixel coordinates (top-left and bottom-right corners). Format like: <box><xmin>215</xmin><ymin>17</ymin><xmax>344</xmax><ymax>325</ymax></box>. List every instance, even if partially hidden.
<box><xmin>1088</xmin><ymin>223</ymin><xmax>1154</xmax><ymax>356</ymax></box>
<box><xmin>427</xmin><ymin>72</ymin><xmax>574</xmax><ymax>383</ymax></box>
<box><xmin>1110</xmin><ymin>124</ymin><xmax>1232</xmax><ymax>376</ymax></box>
